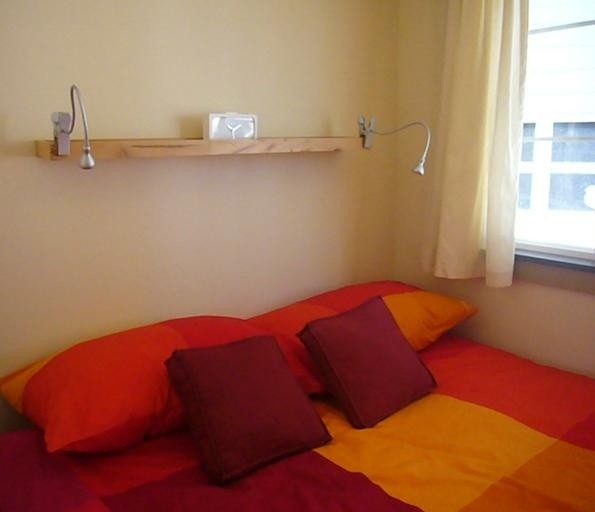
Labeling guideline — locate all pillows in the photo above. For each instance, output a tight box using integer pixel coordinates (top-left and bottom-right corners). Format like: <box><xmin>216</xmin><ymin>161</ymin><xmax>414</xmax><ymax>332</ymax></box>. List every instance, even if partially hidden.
<box><xmin>165</xmin><ymin>337</ymin><xmax>334</xmax><ymax>486</ymax></box>
<box><xmin>0</xmin><ymin>315</ymin><xmax>323</xmax><ymax>455</ymax></box>
<box><xmin>296</xmin><ymin>295</ymin><xmax>437</xmax><ymax>430</ymax></box>
<box><xmin>246</xmin><ymin>280</ymin><xmax>479</xmax><ymax>391</ymax></box>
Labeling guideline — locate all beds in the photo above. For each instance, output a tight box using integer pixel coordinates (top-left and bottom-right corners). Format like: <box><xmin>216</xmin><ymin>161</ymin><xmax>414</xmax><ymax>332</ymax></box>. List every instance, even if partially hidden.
<box><xmin>0</xmin><ymin>280</ymin><xmax>594</xmax><ymax>511</ymax></box>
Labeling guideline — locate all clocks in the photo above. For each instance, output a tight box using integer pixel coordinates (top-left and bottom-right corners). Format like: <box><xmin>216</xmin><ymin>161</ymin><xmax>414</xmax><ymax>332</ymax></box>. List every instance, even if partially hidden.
<box><xmin>202</xmin><ymin>112</ymin><xmax>258</xmax><ymax>139</ymax></box>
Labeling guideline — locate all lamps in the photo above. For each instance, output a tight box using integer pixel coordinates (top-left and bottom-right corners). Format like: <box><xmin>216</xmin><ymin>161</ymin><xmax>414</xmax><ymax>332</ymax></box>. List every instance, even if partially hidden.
<box><xmin>52</xmin><ymin>86</ymin><xmax>96</xmax><ymax>167</ymax></box>
<box><xmin>359</xmin><ymin>114</ymin><xmax>429</xmax><ymax>175</ymax></box>
